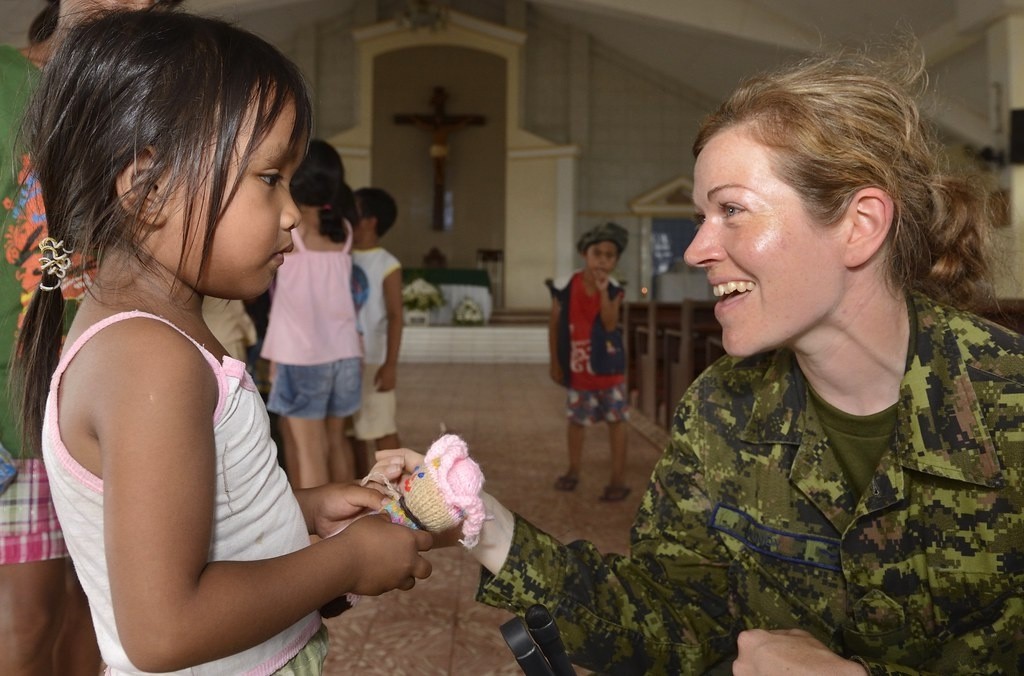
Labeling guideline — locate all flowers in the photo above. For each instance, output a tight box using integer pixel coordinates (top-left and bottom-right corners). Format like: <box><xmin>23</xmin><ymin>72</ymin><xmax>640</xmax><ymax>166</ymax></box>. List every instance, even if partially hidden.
<box><xmin>452</xmin><ymin>297</ymin><xmax>484</xmax><ymax>324</ymax></box>
<box><xmin>401</xmin><ymin>272</ymin><xmax>444</xmax><ymax>313</ymax></box>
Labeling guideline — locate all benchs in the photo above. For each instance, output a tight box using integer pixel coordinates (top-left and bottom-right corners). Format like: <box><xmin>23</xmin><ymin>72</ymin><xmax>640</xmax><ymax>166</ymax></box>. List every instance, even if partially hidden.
<box><xmin>619</xmin><ymin>296</ymin><xmax>1024</xmax><ymax>454</ymax></box>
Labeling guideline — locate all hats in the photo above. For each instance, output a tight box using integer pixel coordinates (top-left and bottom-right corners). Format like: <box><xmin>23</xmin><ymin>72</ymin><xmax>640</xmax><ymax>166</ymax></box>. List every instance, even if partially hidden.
<box><xmin>577</xmin><ymin>222</ymin><xmax>628</xmax><ymax>251</ymax></box>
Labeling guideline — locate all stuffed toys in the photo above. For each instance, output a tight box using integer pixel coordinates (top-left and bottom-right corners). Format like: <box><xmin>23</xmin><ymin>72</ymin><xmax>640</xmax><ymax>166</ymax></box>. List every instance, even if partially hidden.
<box><xmin>327</xmin><ymin>433</ymin><xmax>485</xmax><ymax>551</ymax></box>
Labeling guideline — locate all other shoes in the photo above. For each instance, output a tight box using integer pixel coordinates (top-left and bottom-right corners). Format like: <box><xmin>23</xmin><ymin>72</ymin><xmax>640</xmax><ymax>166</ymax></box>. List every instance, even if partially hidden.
<box><xmin>600</xmin><ymin>486</ymin><xmax>630</xmax><ymax>501</ymax></box>
<box><xmin>555</xmin><ymin>476</ymin><xmax>577</xmax><ymax>491</ymax></box>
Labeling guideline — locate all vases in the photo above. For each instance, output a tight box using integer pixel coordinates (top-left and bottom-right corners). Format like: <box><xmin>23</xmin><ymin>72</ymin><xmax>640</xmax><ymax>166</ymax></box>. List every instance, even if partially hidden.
<box><xmin>403</xmin><ymin>307</ymin><xmax>431</xmax><ymax>327</ymax></box>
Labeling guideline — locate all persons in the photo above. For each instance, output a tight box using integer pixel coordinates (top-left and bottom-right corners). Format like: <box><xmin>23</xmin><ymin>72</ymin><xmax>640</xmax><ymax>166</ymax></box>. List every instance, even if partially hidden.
<box><xmin>0</xmin><ymin>0</ymin><xmax>436</xmax><ymax>676</ymax></box>
<box><xmin>364</xmin><ymin>52</ymin><xmax>1024</xmax><ymax>676</ymax></box>
